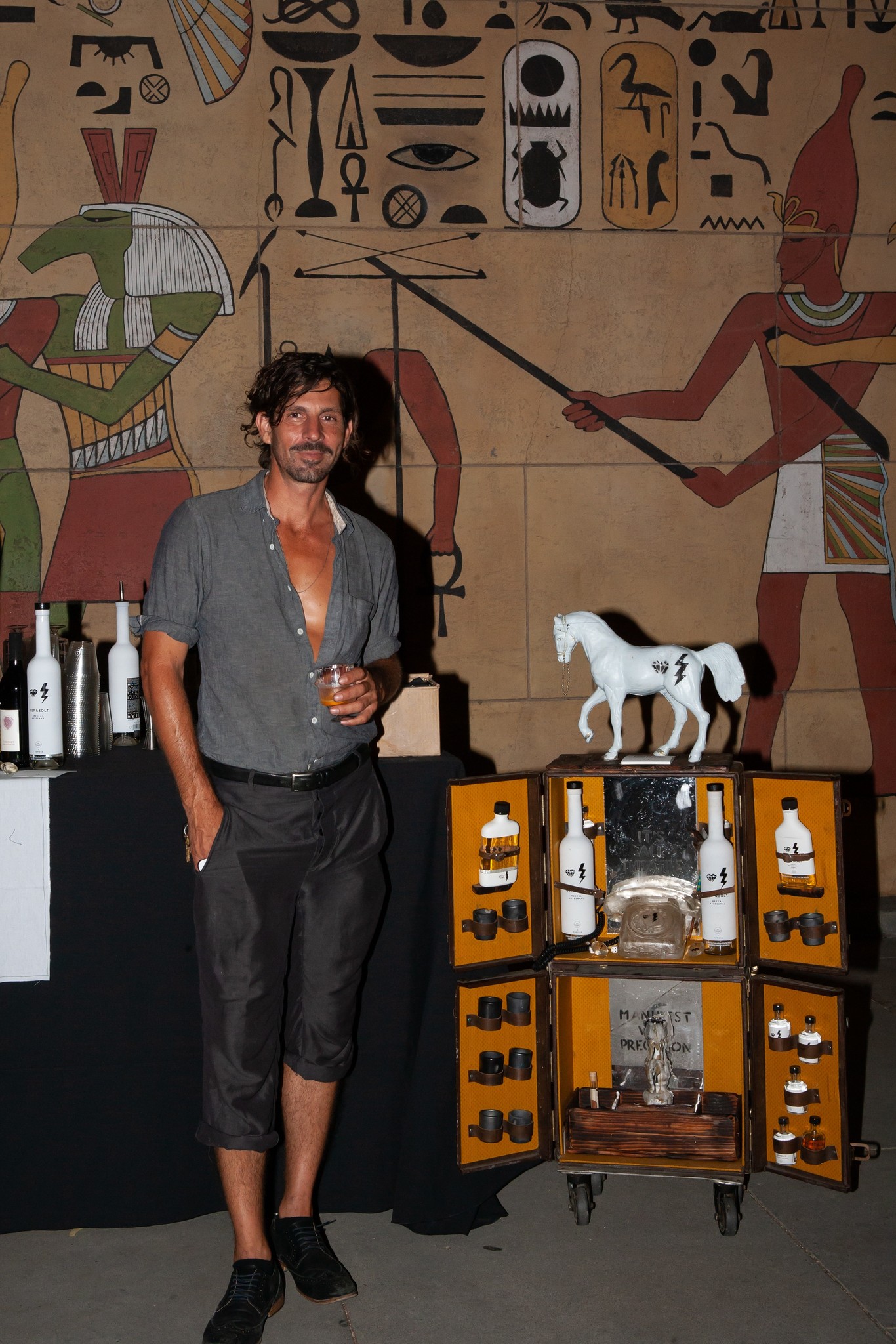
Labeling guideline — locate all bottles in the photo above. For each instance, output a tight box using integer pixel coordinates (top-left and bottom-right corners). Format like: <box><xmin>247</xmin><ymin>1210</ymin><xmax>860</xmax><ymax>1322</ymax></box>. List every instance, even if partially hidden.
<box><xmin>559</xmin><ymin>781</ymin><xmax>597</xmax><ymax>937</ymax></box>
<box><xmin>772</xmin><ymin>1116</ymin><xmax>797</xmax><ymax>1166</ymax></box>
<box><xmin>478</xmin><ymin>801</ymin><xmax>521</xmax><ymax>887</ymax></box>
<box><xmin>784</xmin><ymin>1065</ymin><xmax>808</xmax><ymax>1115</ymax></box>
<box><xmin>0</xmin><ymin>602</ymin><xmax>65</xmax><ymax>771</ymax></box>
<box><xmin>775</xmin><ymin>796</ymin><xmax>816</xmax><ymax>890</ymax></box>
<box><xmin>108</xmin><ymin>600</ymin><xmax>141</xmax><ymax>734</ymax></box>
<box><xmin>798</xmin><ymin>1015</ymin><xmax>823</xmax><ymax>1064</ymax></box>
<box><xmin>801</xmin><ymin>1115</ymin><xmax>825</xmax><ymax>1165</ymax></box>
<box><xmin>768</xmin><ymin>1003</ymin><xmax>791</xmax><ymax>1038</ymax></box>
<box><xmin>699</xmin><ymin>782</ymin><xmax>737</xmax><ymax>956</ymax></box>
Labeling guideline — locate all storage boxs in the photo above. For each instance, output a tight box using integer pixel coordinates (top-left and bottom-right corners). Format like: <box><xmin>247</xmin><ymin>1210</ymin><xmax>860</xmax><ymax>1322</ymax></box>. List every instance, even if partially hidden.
<box><xmin>377</xmin><ymin>674</ymin><xmax>444</xmax><ymax>757</ymax></box>
<box><xmin>562</xmin><ymin>1085</ymin><xmax>741</xmax><ymax>1163</ymax></box>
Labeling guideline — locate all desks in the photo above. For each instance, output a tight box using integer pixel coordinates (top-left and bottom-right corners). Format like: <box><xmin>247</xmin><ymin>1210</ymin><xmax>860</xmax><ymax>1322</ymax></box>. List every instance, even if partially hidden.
<box><xmin>1</xmin><ymin>747</ymin><xmax>544</xmax><ymax>1236</ymax></box>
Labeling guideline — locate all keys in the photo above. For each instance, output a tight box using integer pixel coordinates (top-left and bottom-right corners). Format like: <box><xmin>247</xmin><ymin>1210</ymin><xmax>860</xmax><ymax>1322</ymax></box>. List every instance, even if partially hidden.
<box><xmin>185</xmin><ymin>835</ymin><xmax>191</xmax><ymax>863</ymax></box>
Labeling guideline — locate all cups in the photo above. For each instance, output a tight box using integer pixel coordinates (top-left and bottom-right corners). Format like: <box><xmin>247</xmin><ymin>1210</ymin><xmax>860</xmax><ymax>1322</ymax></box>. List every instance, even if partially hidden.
<box><xmin>479</xmin><ymin>1109</ymin><xmax>504</xmax><ymax>1130</ymax></box>
<box><xmin>315</xmin><ymin>663</ymin><xmax>357</xmax><ymax>705</ymax></box>
<box><xmin>478</xmin><ymin>996</ymin><xmax>503</xmax><ymax>1019</ymax></box>
<box><xmin>63</xmin><ymin>641</ymin><xmax>100</xmax><ymax>760</ymax></box>
<box><xmin>799</xmin><ymin>913</ymin><xmax>825</xmax><ymax>946</ymax></box>
<box><xmin>473</xmin><ymin>908</ymin><xmax>497</xmax><ymax>941</ymax></box>
<box><xmin>479</xmin><ymin>1051</ymin><xmax>504</xmax><ymax>1074</ymax></box>
<box><xmin>508</xmin><ymin>1047</ymin><xmax>533</xmax><ymax>1069</ymax></box>
<box><xmin>506</xmin><ymin>992</ymin><xmax>531</xmax><ymax>1013</ymax></box>
<box><xmin>508</xmin><ymin>1109</ymin><xmax>533</xmax><ymax>1144</ymax></box>
<box><xmin>764</xmin><ymin>910</ymin><xmax>791</xmax><ymax>942</ymax></box>
<box><xmin>501</xmin><ymin>899</ymin><xmax>527</xmax><ymax>920</ymax></box>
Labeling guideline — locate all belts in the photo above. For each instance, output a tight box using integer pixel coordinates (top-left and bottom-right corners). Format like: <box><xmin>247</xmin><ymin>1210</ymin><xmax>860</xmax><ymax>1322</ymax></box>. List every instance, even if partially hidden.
<box><xmin>202</xmin><ymin>743</ymin><xmax>373</xmax><ymax>792</ymax></box>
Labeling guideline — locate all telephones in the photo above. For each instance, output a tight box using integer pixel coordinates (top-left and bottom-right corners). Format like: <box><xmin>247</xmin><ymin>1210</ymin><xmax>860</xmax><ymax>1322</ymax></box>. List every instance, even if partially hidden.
<box><xmin>601</xmin><ymin>877</ymin><xmax>701</xmax><ymax>961</ymax></box>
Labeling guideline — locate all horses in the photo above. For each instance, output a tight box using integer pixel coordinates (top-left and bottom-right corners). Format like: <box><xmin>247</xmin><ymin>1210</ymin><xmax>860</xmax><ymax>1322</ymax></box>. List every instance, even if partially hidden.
<box><xmin>552</xmin><ymin>610</ymin><xmax>746</xmax><ymax>766</ymax></box>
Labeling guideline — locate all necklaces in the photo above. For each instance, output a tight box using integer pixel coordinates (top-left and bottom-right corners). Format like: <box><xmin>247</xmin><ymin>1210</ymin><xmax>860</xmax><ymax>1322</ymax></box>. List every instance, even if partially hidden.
<box><xmin>277</xmin><ymin>508</ymin><xmax>332</xmax><ymax>593</ymax></box>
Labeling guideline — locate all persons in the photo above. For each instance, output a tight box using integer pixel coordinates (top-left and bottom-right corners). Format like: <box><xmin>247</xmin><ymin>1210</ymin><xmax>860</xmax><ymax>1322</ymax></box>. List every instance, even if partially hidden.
<box><xmin>136</xmin><ymin>351</ymin><xmax>405</xmax><ymax>1344</ymax></box>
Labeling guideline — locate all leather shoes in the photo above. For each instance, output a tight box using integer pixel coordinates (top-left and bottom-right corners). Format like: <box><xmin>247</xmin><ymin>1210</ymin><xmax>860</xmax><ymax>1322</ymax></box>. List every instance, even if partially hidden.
<box><xmin>201</xmin><ymin>1245</ymin><xmax>286</xmax><ymax>1344</ymax></box>
<box><xmin>265</xmin><ymin>1208</ymin><xmax>358</xmax><ymax>1304</ymax></box>
